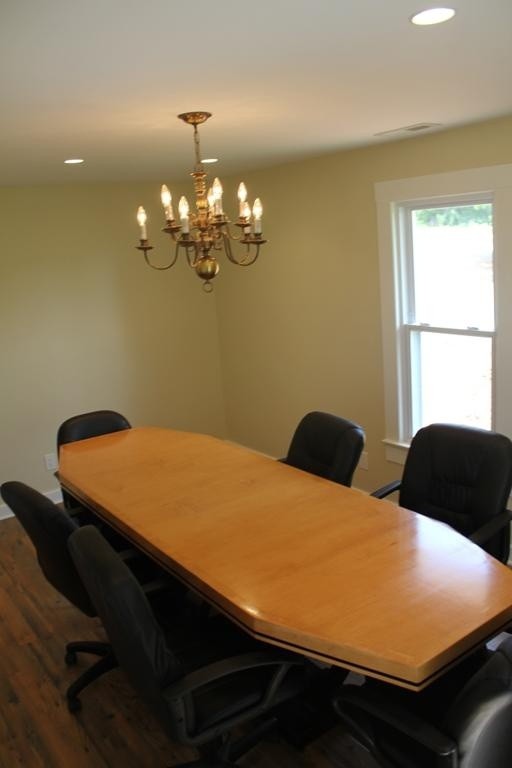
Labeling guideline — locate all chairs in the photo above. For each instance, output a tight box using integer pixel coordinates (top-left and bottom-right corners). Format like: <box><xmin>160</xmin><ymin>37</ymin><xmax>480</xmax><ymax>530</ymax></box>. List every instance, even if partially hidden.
<box><xmin>65</xmin><ymin>523</ymin><xmax>329</xmax><ymax>768</ymax></box>
<box><xmin>0</xmin><ymin>479</ymin><xmax>192</xmax><ymax>714</ymax></box>
<box><xmin>330</xmin><ymin>632</ymin><xmax>511</xmax><ymax>768</ymax></box>
<box><xmin>56</xmin><ymin>408</ymin><xmax>134</xmax><ymax>526</ymax></box>
<box><xmin>370</xmin><ymin>423</ymin><xmax>510</xmax><ymax>569</ymax></box>
<box><xmin>275</xmin><ymin>410</ymin><xmax>366</xmax><ymax>487</ymax></box>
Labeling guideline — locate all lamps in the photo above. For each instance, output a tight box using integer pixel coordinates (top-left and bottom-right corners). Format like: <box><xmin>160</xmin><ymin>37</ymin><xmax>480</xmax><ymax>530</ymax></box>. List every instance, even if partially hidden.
<box><xmin>134</xmin><ymin>111</ymin><xmax>271</xmax><ymax>296</ymax></box>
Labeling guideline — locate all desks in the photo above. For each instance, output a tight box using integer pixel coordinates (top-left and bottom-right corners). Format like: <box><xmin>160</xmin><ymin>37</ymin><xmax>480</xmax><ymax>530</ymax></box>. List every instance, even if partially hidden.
<box><xmin>55</xmin><ymin>426</ymin><xmax>511</xmax><ymax>693</ymax></box>
